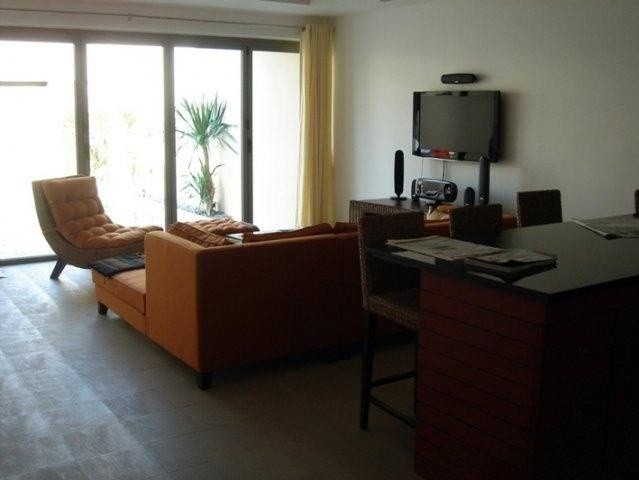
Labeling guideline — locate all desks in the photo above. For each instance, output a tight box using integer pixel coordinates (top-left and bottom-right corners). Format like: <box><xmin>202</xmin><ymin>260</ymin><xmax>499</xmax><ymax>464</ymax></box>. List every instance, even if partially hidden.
<box><xmin>411</xmin><ymin>214</ymin><xmax>638</xmax><ymax>479</ymax></box>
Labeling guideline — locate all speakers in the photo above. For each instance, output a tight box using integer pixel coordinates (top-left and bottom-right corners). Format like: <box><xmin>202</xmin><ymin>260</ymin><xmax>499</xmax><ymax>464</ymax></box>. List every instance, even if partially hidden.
<box><xmin>411</xmin><ymin>179</ymin><xmax>419</xmax><ymax>199</ymax></box>
<box><xmin>464</xmin><ymin>187</ymin><xmax>476</xmax><ymax>206</ymax></box>
<box><xmin>478</xmin><ymin>155</ymin><xmax>491</xmax><ymax>204</ymax></box>
<box><xmin>390</xmin><ymin>150</ymin><xmax>406</xmax><ymax>201</ymax></box>
<box><xmin>441</xmin><ymin>74</ymin><xmax>477</xmax><ymax>84</ymax></box>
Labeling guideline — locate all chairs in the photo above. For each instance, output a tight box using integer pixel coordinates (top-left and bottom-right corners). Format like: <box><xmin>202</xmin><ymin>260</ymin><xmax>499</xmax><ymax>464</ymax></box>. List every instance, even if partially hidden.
<box><xmin>31</xmin><ymin>174</ymin><xmax>163</xmax><ymax>279</ymax></box>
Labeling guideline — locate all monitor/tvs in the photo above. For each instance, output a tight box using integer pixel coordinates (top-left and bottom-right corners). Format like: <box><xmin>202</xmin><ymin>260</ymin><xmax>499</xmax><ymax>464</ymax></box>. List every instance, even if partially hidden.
<box><xmin>412</xmin><ymin>89</ymin><xmax>502</xmax><ymax>162</ymax></box>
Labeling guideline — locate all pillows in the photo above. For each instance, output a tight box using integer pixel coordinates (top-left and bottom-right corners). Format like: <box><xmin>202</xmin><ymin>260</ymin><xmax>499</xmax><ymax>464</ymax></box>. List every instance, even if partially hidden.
<box><xmin>167</xmin><ymin>221</ymin><xmax>358</xmax><ymax>248</ymax></box>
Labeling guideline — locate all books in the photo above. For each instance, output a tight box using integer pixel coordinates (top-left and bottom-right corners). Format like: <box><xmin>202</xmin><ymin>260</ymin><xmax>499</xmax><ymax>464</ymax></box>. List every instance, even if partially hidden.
<box><xmin>386</xmin><ymin>235</ymin><xmax>501</xmax><ymax>276</ymax></box>
<box><xmin>463</xmin><ymin>248</ymin><xmax>558</xmax><ymax>274</ymax></box>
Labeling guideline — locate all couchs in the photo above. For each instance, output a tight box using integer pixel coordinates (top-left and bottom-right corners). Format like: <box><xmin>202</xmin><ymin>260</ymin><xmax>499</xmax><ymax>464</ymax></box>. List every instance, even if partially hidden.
<box><xmin>90</xmin><ymin>198</ymin><xmax>426</xmax><ymax>391</ymax></box>
